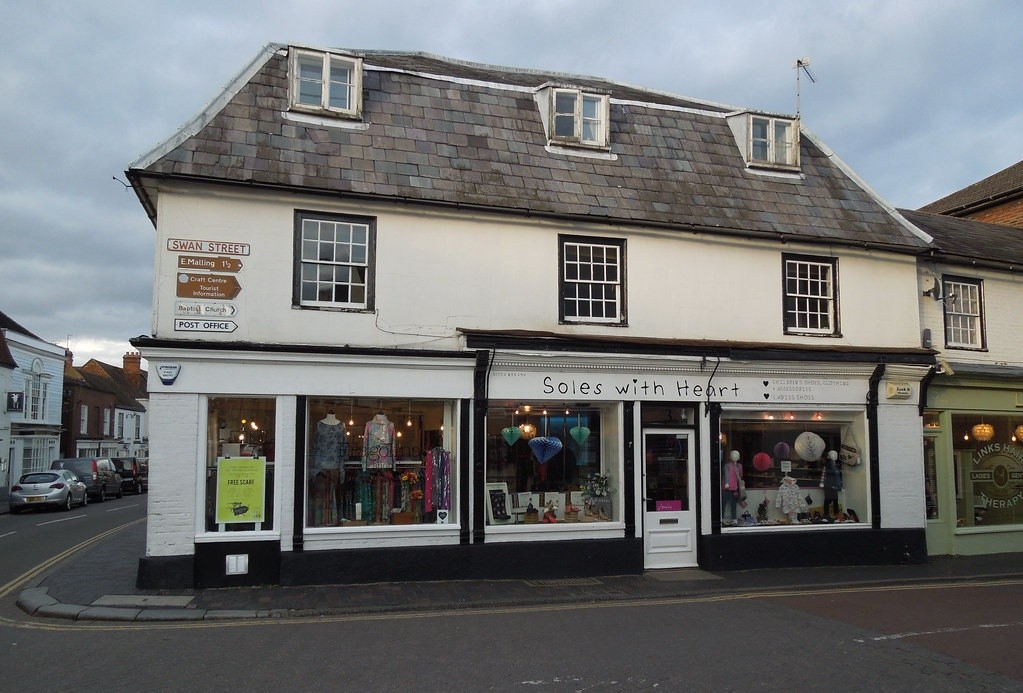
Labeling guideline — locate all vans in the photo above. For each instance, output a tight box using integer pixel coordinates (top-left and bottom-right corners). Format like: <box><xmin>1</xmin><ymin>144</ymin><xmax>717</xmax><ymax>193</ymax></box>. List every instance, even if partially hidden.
<box><xmin>110</xmin><ymin>456</ymin><xmax>148</xmax><ymax>494</ymax></box>
<box><xmin>51</xmin><ymin>456</ymin><xmax>125</xmax><ymax>503</ymax></box>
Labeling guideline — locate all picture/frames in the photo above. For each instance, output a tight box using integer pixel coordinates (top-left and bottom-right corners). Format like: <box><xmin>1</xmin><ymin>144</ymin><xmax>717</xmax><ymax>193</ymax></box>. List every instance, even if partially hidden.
<box><xmin>486</xmin><ymin>482</ymin><xmax>515</xmax><ymax>525</ymax></box>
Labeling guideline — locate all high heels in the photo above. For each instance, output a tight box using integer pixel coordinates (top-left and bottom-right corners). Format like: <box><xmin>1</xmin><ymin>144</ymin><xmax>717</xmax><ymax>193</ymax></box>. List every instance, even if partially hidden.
<box><xmin>598</xmin><ymin>507</ymin><xmax>609</xmax><ymax>520</ymax></box>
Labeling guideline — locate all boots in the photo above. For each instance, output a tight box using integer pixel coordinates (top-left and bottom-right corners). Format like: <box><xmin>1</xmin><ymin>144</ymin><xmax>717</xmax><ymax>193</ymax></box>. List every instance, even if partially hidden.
<box><xmin>490</xmin><ymin>492</ymin><xmax>511</xmax><ymax>520</ymax></box>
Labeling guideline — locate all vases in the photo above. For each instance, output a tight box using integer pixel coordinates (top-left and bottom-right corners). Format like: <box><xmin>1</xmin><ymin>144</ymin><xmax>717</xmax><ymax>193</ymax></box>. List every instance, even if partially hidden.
<box><xmin>757</xmin><ymin>515</ymin><xmax>768</xmax><ymax>523</ymax></box>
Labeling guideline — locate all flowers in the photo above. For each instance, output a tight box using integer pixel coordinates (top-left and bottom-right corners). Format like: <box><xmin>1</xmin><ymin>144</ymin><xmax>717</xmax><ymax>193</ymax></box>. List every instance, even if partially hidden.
<box><xmin>757</xmin><ymin>496</ymin><xmax>770</xmax><ymax>516</ymax></box>
<box><xmin>579</xmin><ymin>469</ymin><xmax>618</xmax><ymax>498</ymax></box>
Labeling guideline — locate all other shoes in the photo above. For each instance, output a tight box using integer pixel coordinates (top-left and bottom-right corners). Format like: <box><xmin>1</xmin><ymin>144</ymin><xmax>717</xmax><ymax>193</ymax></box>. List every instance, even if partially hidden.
<box><xmin>543</xmin><ymin>500</ymin><xmax>553</xmax><ymax>513</ymax></box>
<box><xmin>572</xmin><ymin>506</ymin><xmax>581</xmax><ymax>512</ymax></box>
<box><xmin>565</xmin><ymin>505</ymin><xmax>572</xmax><ymax>514</ymax></box>
<box><xmin>722</xmin><ymin>512</ymin><xmax>859</xmax><ymax>526</ymax></box>
<box><xmin>533</xmin><ymin>507</ymin><xmax>538</xmax><ymax>513</ymax></box>
<box><xmin>526</xmin><ymin>503</ymin><xmax>533</xmax><ymax>515</ymax></box>
<box><xmin>546</xmin><ymin>502</ymin><xmax>559</xmax><ymax>511</ymax></box>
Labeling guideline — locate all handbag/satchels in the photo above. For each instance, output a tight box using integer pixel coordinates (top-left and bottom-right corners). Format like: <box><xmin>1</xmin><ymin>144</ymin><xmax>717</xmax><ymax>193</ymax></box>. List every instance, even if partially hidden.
<box><xmin>839</xmin><ymin>425</ymin><xmax>861</xmax><ymax>466</ymax></box>
<box><xmin>738</xmin><ymin>480</ymin><xmax>747</xmax><ymax>501</ymax></box>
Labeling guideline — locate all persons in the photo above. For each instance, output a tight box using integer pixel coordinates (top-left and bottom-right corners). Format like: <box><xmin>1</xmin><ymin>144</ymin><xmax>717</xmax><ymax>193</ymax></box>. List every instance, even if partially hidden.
<box><xmin>312</xmin><ymin>414</ymin><xmax>349</xmax><ymax>483</ymax></box>
<box><xmin>721</xmin><ymin>451</ymin><xmax>743</xmax><ymax>525</ymax></box>
<box><xmin>362</xmin><ymin>413</ymin><xmax>396</xmax><ymax>471</ymax></box>
<box><xmin>925</xmin><ymin>478</ymin><xmax>936</xmax><ymax>519</ymax></box>
<box><xmin>648</xmin><ymin>462</ymin><xmax>670</xmax><ymax>500</ymax></box>
<box><xmin>819</xmin><ymin>450</ymin><xmax>842</xmax><ymax>522</ymax></box>
<box><xmin>514</xmin><ymin>438</ymin><xmax>577</xmax><ymax>491</ymax></box>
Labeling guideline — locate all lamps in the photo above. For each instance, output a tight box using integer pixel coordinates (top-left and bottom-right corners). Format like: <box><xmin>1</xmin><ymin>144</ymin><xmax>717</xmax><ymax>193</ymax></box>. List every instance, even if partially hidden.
<box><xmin>518</xmin><ymin>405</ymin><xmax>537</xmax><ymax>439</ymax></box>
<box><xmin>1015</xmin><ymin>425</ymin><xmax>1023</xmax><ymax>442</ymax></box>
<box><xmin>971</xmin><ymin>417</ymin><xmax>995</xmax><ymax>443</ymax></box>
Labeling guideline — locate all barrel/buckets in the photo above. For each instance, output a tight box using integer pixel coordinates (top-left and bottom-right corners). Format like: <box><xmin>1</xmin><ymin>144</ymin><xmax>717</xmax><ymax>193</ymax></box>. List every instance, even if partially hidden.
<box><xmin>565</xmin><ymin>511</ymin><xmax>578</xmax><ymax>523</ymax></box>
<box><xmin>524</xmin><ymin>512</ymin><xmax>538</xmax><ymax>523</ymax></box>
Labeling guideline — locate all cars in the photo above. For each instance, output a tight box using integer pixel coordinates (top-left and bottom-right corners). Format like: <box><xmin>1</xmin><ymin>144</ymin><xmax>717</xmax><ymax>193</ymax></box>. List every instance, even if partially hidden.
<box><xmin>9</xmin><ymin>469</ymin><xmax>88</xmax><ymax>515</ymax></box>
<box><xmin>925</xmin><ymin>477</ymin><xmax>990</xmax><ymax>524</ymax></box>
